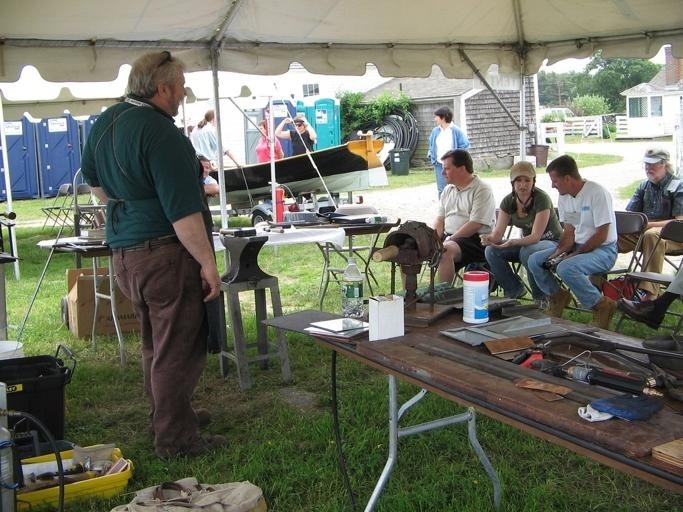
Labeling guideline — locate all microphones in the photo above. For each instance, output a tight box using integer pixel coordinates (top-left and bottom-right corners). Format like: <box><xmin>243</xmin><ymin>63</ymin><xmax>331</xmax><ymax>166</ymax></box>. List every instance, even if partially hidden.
<box><xmin>543</xmin><ymin>251</ymin><xmax>567</xmax><ymax>269</ymax></box>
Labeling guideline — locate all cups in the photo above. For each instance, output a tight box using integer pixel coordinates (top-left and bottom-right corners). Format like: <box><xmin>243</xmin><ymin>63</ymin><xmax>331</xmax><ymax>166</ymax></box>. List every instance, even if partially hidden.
<box><xmin>271</xmin><ymin>188</ymin><xmax>285</xmax><ymax>227</ymax></box>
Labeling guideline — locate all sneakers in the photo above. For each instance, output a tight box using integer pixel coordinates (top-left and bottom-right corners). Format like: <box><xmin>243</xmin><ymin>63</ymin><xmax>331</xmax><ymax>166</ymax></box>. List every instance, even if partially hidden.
<box><xmin>155</xmin><ymin>407</ymin><xmax>227</xmax><ymax>458</ymax></box>
<box><xmin>592</xmin><ymin>297</ymin><xmax>616</xmax><ymax>328</ymax></box>
<box><xmin>505</xmin><ymin>289</ymin><xmax>571</xmax><ymax>317</ymax></box>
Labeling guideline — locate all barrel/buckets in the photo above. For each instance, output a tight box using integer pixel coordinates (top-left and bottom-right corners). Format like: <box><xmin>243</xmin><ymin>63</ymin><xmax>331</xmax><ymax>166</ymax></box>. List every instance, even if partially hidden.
<box><xmin>0</xmin><ymin>338</ymin><xmax>23</xmax><ymax>359</ymax></box>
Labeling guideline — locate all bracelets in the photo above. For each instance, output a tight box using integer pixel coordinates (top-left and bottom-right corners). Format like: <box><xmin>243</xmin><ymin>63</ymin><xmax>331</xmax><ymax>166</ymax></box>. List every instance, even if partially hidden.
<box><xmin>305</xmin><ymin>122</ymin><xmax>309</xmax><ymax>129</ymax></box>
<box><xmin>445</xmin><ymin>235</ymin><xmax>453</xmax><ymax>241</ymax></box>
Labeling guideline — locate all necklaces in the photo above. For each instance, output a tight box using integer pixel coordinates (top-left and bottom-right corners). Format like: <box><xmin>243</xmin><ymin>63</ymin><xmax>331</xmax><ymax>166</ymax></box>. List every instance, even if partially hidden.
<box><xmin>516</xmin><ymin>193</ymin><xmax>533</xmax><ymax>215</ymax></box>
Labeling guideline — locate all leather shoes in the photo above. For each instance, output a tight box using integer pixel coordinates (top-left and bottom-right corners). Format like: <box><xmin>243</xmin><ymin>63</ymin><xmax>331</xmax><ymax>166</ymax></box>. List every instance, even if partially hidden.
<box><xmin>620</xmin><ymin>297</ymin><xmax>665</xmax><ymax>328</ymax></box>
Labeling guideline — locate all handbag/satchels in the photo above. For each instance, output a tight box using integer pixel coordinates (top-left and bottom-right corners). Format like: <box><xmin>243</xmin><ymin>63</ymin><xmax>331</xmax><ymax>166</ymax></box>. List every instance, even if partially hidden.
<box><xmin>112</xmin><ymin>477</ymin><xmax>267</xmax><ymax>512</ymax></box>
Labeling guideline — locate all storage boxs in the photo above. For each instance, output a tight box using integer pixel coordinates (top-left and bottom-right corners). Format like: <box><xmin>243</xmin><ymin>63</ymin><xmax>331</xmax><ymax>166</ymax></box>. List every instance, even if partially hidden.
<box><xmin>0</xmin><ymin>344</ymin><xmax>76</xmax><ymax>445</ymax></box>
<box><xmin>66</xmin><ymin>268</ymin><xmax>142</xmax><ymax>337</ymax></box>
<box><xmin>16</xmin><ymin>443</ymin><xmax>132</xmax><ymax>509</ymax></box>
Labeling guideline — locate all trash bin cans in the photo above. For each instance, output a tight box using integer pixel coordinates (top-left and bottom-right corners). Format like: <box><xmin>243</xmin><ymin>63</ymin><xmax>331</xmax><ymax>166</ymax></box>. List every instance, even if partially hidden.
<box><xmin>388</xmin><ymin>148</ymin><xmax>410</xmax><ymax>175</ymax></box>
<box><xmin>531</xmin><ymin>144</ymin><xmax>549</xmax><ymax>168</ymax></box>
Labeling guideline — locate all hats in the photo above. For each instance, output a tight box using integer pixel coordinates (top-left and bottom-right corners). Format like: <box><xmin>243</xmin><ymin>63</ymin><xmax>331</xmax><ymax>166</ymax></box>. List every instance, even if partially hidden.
<box><xmin>510</xmin><ymin>161</ymin><xmax>535</xmax><ymax>181</ymax></box>
<box><xmin>644</xmin><ymin>150</ymin><xmax>670</xmax><ymax>164</ymax></box>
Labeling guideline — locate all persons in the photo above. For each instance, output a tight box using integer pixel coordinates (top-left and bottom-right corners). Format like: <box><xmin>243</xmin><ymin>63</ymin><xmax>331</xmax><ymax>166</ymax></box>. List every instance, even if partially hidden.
<box><xmin>193</xmin><ymin>152</ymin><xmax>221</xmax><ymax>195</ymax></box>
<box><xmin>427</xmin><ymin>106</ymin><xmax>469</xmax><ymax>203</ymax></box>
<box><xmin>615</xmin><ymin>146</ymin><xmax>682</xmax><ymax>301</ymax></box>
<box><xmin>79</xmin><ymin>49</ymin><xmax>231</xmax><ymax>458</ymax></box>
<box><xmin>253</xmin><ymin>120</ymin><xmax>282</xmax><ymax>164</ymax></box>
<box><xmin>525</xmin><ymin>155</ymin><xmax>620</xmax><ymax>329</ymax></box>
<box><xmin>477</xmin><ymin>160</ymin><xmax>565</xmax><ymax>314</ymax></box>
<box><xmin>274</xmin><ymin>115</ymin><xmax>317</xmax><ymax>158</ymax></box>
<box><xmin>620</xmin><ymin>254</ymin><xmax>682</xmax><ymax>333</ymax></box>
<box><xmin>431</xmin><ymin>149</ymin><xmax>496</xmax><ymax>291</ymax></box>
<box><xmin>191</xmin><ymin>109</ymin><xmax>229</xmax><ymax>169</ymax></box>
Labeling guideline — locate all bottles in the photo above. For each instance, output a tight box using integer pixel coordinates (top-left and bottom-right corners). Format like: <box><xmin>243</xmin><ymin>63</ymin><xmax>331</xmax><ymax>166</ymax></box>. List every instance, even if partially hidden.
<box><xmin>340</xmin><ymin>258</ymin><xmax>363</xmax><ymax>323</ymax></box>
<box><xmin>365</xmin><ymin>215</ymin><xmax>398</xmax><ymax>225</ymax></box>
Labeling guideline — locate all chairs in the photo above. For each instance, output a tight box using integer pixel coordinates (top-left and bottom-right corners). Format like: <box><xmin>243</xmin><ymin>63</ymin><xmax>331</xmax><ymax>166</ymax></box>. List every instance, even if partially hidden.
<box><xmin>38</xmin><ymin>184</ymin><xmax>104</xmax><ymax>235</ymax></box>
<box><xmin>420</xmin><ymin>208</ymin><xmax>683</xmax><ymax>335</ymax></box>
<box><xmin>316</xmin><ymin>240</ymin><xmax>380</xmax><ymax>296</ymax></box>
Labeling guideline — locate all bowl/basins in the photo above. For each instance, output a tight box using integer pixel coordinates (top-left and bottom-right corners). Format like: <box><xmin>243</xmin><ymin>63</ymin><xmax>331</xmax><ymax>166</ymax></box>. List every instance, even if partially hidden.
<box><xmin>642</xmin><ymin>335</ymin><xmax>683</xmax><ymax>370</ymax></box>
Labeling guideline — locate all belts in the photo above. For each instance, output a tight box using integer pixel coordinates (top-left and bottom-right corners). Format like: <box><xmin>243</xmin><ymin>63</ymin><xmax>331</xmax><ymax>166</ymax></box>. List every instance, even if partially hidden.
<box><xmin>113</xmin><ymin>236</ymin><xmax>178</xmax><ymax>251</ymax></box>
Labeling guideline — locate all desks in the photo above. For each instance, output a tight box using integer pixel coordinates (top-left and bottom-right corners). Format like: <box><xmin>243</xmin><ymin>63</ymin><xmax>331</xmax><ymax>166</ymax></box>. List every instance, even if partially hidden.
<box><xmin>315</xmin><ymin>310</ymin><xmax>683</xmax><ymax>512</ymax></box>
<box><xmin>42</xmin><ymin>223</ymin><xmax>389</xmax><ymax>371</ymax></box>
